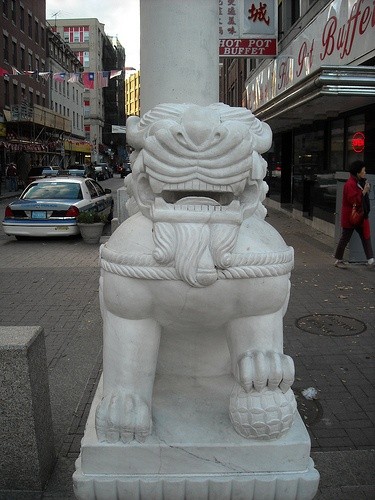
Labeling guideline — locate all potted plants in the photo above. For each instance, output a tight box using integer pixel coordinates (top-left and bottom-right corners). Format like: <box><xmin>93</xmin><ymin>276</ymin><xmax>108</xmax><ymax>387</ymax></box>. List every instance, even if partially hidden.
<box><xmin>76</xmin><ymin>209</ymin><xmax>108</xmax><ymax>244</ymax></box>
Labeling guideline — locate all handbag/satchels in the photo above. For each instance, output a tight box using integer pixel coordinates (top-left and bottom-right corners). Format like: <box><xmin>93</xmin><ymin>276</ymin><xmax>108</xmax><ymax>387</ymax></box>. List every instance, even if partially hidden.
<box><xmin>349</xmin><ymin>203</ymin><xmax>363</xmax><ymax>224</ymax></box>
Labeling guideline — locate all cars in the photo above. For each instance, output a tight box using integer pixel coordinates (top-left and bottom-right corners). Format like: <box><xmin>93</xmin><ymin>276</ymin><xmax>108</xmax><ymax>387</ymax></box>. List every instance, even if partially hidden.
<box><xmin>120</xmin><ymin>161</ymin><xmax>132</xmax><ymax>179</ymax></box>
<box><xmin>0</xmin><ymin>174</ymin><xmax>114</xmax><ymax>241</ymax></box>
<box><xmin>23</xmin><ymin>161</ymin><xmax>114</xmax><ymax>188</ymax></box>
<box><xmin>266</xmin><ymin>163</ymin><xmax>281</xmax><ymax>180</ymax></box>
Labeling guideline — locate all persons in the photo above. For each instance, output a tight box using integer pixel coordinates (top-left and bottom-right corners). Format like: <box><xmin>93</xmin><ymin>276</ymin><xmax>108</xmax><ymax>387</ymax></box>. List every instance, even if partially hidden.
<box><xmin>5</xmin><ymin>163</ymin><xmax>17</xmax><ymax>192</ymax></box>
<box><xmin>334</xmin><ymin>160</ymin><xmax>375</xmax><ymax>269</ymax></box>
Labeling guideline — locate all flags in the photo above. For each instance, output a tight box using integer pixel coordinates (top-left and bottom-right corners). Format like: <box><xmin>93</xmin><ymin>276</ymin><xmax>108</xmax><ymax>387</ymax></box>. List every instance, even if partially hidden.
<box><xmin>82</xmin><ymin>72</ymin><xmax>94</xmax><ymax>89</ymax></box>
<box><xmin>110</xmin><ymin>70</ymin><xmax>122</xmax><ymax>79</ymax></box>
<box><xmin>123</xmin><ymin>66</ymin><xmax>135</xmax><ymax>70</ymax></box>
<box><xmin>0</xmin><ymin>68</ymin><xmax>9</xmax><ymax>76</ymax></box>
<box><xmin>97</xmin><ymin>71</ymin><xmax>109</xmax><ymax>87</ymax></box>
<box><xmin>12</xmin><ymin>67</ymin><xmax>51</xmax><ymax>81</ymax></box>
<box><xmin>52</xmin><ymin>72</ymin><xmax>81</xmax><ymax>83</ymax></box>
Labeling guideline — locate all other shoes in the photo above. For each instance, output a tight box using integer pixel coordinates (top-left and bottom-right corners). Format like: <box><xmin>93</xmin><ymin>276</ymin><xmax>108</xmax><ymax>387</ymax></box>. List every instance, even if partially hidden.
<box><xmin>366</xmin><ymin>258</ymin><xmax>375</xmax><ymax>269</ymax></box>
<box><xmin>334</xmin><ymin>260</ymin><xmax>348</xmax><ymax>269</ymax></box>
